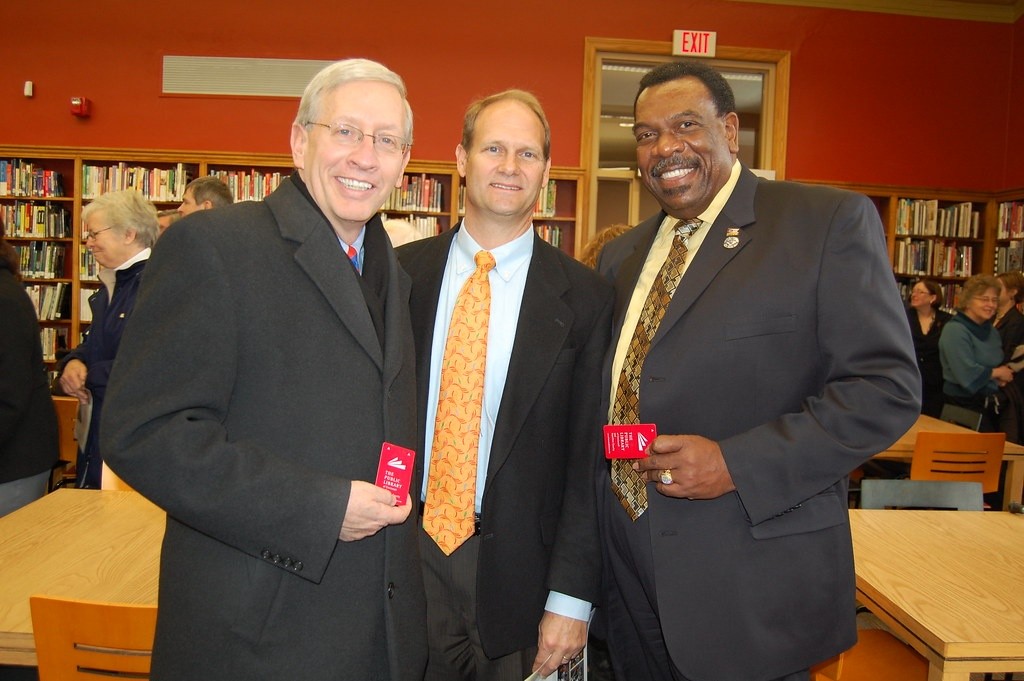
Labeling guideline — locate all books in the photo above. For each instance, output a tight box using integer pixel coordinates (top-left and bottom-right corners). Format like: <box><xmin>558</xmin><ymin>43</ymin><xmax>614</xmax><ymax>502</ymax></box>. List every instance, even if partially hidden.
<box><xmin>378</xmin><ymin>174</ymin><xmax>466</xmax><ymax>247</ymax></box>
<box><xmin>0</xmin><ymin>158</ymin><xmax>292</xmax><ymax>388</ymax></box>
<box><xmin>894</xmin><ymin>198</ymin><xmax>1024</xmax><ymax>316</ymax></box>
<box><xmin>533</xmin><ymin>180</ymin><xmax>561</xmax><ymax>249</ymax></box>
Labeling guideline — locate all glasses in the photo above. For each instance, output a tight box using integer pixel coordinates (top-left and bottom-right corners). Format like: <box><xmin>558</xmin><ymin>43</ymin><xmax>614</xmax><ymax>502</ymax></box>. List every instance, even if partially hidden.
<box><xmin>911</xmin><ymin>290</ymin><xmax>928</xmax><ymax>295</ymax></box>
<box><xmin>87</xmin><ymin>228</ymin><xmax>111</xmax><ymax>240</ymax></box>
<box><xmin>973</xmin><ymin>296</ymin><xmax>1000</xmax><ymax>305</ymax></box>
<box><xmin>307</xmin><ymin>122</ymin><xmax>412</xmax><ymax>159</ymax></box>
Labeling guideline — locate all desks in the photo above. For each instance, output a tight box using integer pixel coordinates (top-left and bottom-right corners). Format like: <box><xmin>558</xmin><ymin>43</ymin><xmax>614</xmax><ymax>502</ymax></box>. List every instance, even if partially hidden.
<box><xmin>849</xmin><ymin>413</ymin><xmax>1023</xmax><ymax>517</ymax></box>
<box><xmin>850</xmin><ymin>502</ymin><xmax>1024</xmax><ymax>681</ymax></box>
<box><xmin>0</xmin><ymin>484</ymin><xmax>208</xmax><ymax>664</ymax></box>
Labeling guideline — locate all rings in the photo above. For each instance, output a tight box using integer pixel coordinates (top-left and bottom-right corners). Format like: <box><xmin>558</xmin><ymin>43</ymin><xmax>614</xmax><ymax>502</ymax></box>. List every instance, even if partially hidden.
<box><xmin>659</xmin><ymin>469</ymin><xmax>673</xmax><ymax>485</ymax></box>
<box><xmin>563</xmin><ymin>656</ymin><xmax>570</xmax><ymax>660</ymax></box>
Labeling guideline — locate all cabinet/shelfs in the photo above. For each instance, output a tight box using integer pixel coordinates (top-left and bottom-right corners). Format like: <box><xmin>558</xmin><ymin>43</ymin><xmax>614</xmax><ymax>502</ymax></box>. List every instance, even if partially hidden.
<box><xmin>0</xmin><ymin>142</ymin><xmax>586</xmax><ymax>383</ymax></box>
<box><xmin>983</xmin><ymin>188</ymin><xmax>1024</xmax><ymax>294</ymax></box>
<box><xmin>801</xmin><ymin>181</ymin><xmax>988</xmax><ymax>304</ymax></box>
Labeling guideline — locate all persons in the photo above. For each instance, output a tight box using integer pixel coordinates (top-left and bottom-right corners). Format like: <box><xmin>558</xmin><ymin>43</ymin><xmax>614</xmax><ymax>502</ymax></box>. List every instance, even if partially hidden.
<box><xmin>906</xmin><ymin>280</ymin><xmax>953</xmax><ymax>420</ymax></box>
<box><xmin>583</xmin><ymin>224</ymin><xmax>632</xmax><ymax>271</ymax></box>
<box><xmin>156</xmin><ymin>208</ymin><xmax>181</xmax><ymax>235</ymax></box>
<box><xmin>939</xmin><ymin>274</ymin><xmax>1013</xmax><ymax>503</ymax></box>
<box><xmin>99</xmin><ymin>57</ymin><xmax>428</xmax><ymax>681</ymax></box>
<box><xmin>989</xmin><ymin>270</ymin><xmax>1024</xmax><ymax>510</ymax></box>
<box><xmin>0</xmin><ymin>216</ymin><xmax>59</xmax><ymax>516</ymax></box>
<box><xmin>594</xmin><ymin>62</ymin><xmax>921</xmax><ymax>681</ymax></box>
<box><xmin>59</xmin><ymin>190</ymin><xmax>160</xmax><ymax>491</ymax></box>
<box><xmin>392</xmin><ymin>89</ymin><xmax>618</xmax><ymax>681</ymax></box>
<box><xmin>179</xmin><ymin>176</ymin><xmax>233</xmax><ymax>218</ymax></box>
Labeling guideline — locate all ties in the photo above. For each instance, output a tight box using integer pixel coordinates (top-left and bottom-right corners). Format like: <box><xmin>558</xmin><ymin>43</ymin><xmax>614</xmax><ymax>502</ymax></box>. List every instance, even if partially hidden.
<box><xmin>347</xmin><ymin>246</ymin><xmax>359</xmax><ymax>273</ymax></box>
<box><xmin>422</xmin><ymin>251</ymin><xmax>496</xmax><ymax>556</ymax></box>
<box><xmin>610</xmin><ymin>218</ymin><xmax>704</xmax><ymax>522</ymax></box>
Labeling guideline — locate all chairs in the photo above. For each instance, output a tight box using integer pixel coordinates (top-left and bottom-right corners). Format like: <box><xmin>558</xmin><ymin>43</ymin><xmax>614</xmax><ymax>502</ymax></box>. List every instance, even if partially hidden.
<box><xmin>912</xmin><ymin>432</ymin><xmax>1009</xmax><ymax>512</ymax></box>
<box><xmin>860</xmin><ymin>479</ymin><xmax>984</xmax><ymax>516</ymax></box>
<box><xmin>939</xmin><ymin>404</ymin><xmax>984</xmax><ymax>430</ymax></box>
<box><xmin>30</xmin><ymin>592</ymin><xmax>164</xmax><ymax>681</ymax></box>
